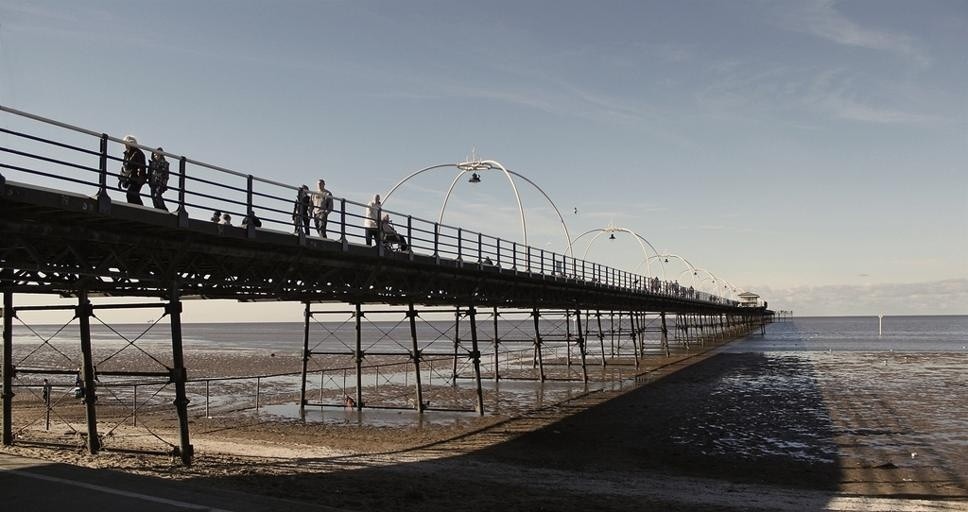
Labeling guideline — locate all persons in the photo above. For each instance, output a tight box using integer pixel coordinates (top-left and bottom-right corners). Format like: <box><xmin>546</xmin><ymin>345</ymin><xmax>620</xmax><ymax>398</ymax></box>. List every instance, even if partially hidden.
<box><xmin>365</xmin><ymin>194</ymin><xmax>382</xmax><ymax>246</ymax></box>
<box><xmin>292</xmin><ymin>184</ymin><xmax>313</xmax><ymax>236</ymax></box>
<box><xmin>147</xmin><ymin>146</ymin><xmax>170</xmax><ymax>210</ymax></box>
<box><xmin>41</xmin><ymin>378</ymin><xmax>50</xmax><ymax>405</ymax></box>
<box><xmin>121</xmin><ymin>134</ymin><xmax>146</xmax><ymax>205</ymax></box>
<box><xmin>309</xmin><ymin>179</ymin><xmax>334</xmax><ymax>239</ymax></box>
<box><xmin>382</xmin><ymin>214</ymin><xmax>414</xmax><ymax>253</ymax></box>
<box><xmin>649</xmin><ymin>277</ymin><xmax>695</xmax><ymax>298</ymax></box>
